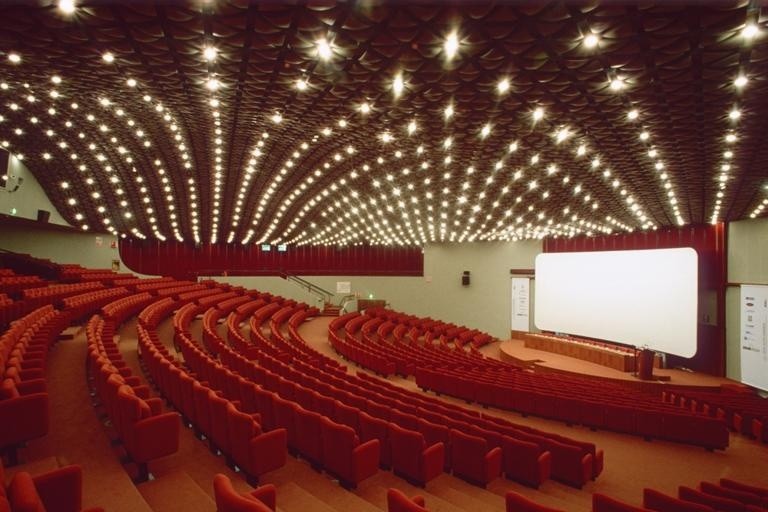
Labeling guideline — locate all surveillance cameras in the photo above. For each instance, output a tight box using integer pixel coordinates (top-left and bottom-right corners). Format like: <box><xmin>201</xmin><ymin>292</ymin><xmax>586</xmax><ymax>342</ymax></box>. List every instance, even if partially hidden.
<box><xmin>10</xmin><ymin>174</ymin><xmax>16</xmax><ymax>180</ymax></box>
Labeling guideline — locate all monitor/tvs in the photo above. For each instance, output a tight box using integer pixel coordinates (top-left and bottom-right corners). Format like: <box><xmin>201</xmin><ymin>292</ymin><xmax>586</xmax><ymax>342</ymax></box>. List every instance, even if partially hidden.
<box><xmin>276</xmin><ymin>244</ymin><xmax>288</xmax><ymax>253</ymax></box>
<box><xmin>260</xmin><ymin>243</ymin><xmax>272</xmax><ymax>252</ymax></box>
